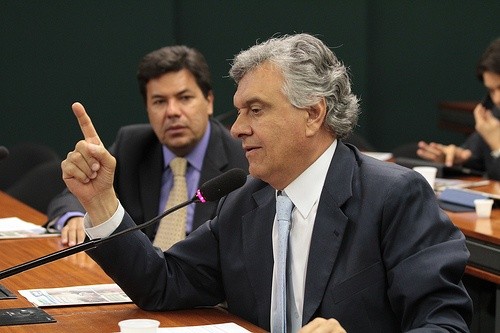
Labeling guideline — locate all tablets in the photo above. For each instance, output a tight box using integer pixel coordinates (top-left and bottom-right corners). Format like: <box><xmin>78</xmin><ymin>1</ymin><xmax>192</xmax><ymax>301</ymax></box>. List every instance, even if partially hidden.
<box><xmin>396</xmin><ymin>158</ymin><xmax>486</xmax><ymax>177</ymax></box>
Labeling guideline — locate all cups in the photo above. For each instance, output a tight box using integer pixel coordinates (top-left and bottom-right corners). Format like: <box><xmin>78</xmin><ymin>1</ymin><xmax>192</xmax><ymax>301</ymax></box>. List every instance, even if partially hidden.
<box><xmin>413</xmin><ymin>166</ymin><xmax>438</xmax><ymax>191</ymax></box>
<box><xmin>474</xmin><ymin>200</ymin><xmax>493</xmax><ymax>218</ymax></box>
<box><xmin>118</xmin><ymin>319</ymin><xmax>160</xmax><ymax>332</ymax></box>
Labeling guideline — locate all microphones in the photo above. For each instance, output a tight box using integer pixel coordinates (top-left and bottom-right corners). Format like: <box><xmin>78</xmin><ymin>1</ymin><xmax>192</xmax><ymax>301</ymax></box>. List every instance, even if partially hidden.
<box><xmin>0</xmin><ymin>169</ymin><xmax>247</xmax><ymax>279</ymax></box>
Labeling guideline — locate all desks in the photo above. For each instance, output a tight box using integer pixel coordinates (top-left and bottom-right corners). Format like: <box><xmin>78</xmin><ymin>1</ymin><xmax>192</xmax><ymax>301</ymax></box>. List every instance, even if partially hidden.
<box><xmin>0</xmin><ymin>192</ymin><xmax>267</xmax><ymax>333</ymax></box>
<box><xmin>362</xmin><ymin>151</ymin><xmax>500</xmax><ymax>282</ymax></box>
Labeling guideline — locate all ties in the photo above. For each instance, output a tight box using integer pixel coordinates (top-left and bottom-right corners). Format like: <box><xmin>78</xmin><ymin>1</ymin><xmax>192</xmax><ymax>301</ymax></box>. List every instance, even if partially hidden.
<box><xmin>153</xmin><ymin>158</ymin><xmax>188</xmax><ymax>252</ymax></box>
<box><xmin>271</xmin><ymin>197</ymin><xmax>293</xmax><ymax>333</ymax></box>
